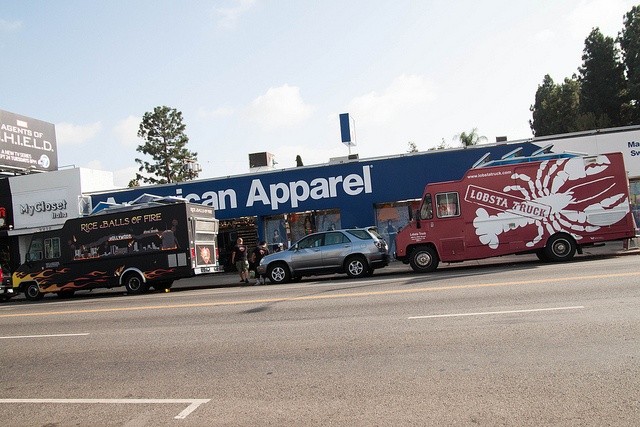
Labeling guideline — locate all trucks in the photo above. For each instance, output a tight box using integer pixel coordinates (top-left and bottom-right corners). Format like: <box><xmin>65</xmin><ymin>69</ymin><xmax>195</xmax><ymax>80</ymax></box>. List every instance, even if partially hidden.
<box><xmin>393</xmin><ymin>152</ymin><xmax>636</xmax><ymax>272</ymax></box>
<box><xmin>12</xmin><ymin>202</ymin><xmax>220</xmax><ymax>301</ymax></box>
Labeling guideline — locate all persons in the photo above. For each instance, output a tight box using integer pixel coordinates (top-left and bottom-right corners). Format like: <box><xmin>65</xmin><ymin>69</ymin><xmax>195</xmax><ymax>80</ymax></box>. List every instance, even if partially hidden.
<box><xmin>304</xmin><ymin>216</ymin><xmax>313</xmax><ymax>235</ymax></box>
<box><xmin>384</xmin><ymin>219</ymin><xmax>398</xmax><ymax>254</ymax></box>
<box><xmin>232</xmin><ymin>237</ymin><xmax>249</xmax><ymax>283</ymax></box>
<box><xmin>328</xmin><ymin>225</ymin><xmax>336</xmax><ymax>230</ymax></box>
<box><xmin>272</xmin><ymin>230</ymin><xmax>281</xmax><ymax>244</ymax></box>
<box><xmin>252</xmin><ymin>241</ymin><xmax>271</xmax><ymax>285</ymax></box>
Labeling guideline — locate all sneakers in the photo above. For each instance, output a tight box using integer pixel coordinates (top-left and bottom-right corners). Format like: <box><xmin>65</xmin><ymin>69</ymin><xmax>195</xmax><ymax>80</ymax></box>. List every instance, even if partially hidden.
<box><xmin>245</xmin><ymin>279</ymin><xmax>249</xmax><ymax>283</ymax></box>
<box><xmin>254</xmin><ymin>282</ymin><xmax>260</xmax><ymax>286</ymax></box>
<box><xmin>240</xmin><ymin>279</ymin><xmax>244</xmax><ymax>282</ymax></box>
<box><xmin>259</xmin><ymin>241</ymin><xmax>265</xmax><ymax>245</ymax></box>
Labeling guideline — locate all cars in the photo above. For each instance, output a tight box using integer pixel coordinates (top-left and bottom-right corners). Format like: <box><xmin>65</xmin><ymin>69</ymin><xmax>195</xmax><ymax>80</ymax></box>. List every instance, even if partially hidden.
<box><xmin>257</xmin><ymin>226</ymin><xmax>390</xmax><ymax>284</ymax></box>
